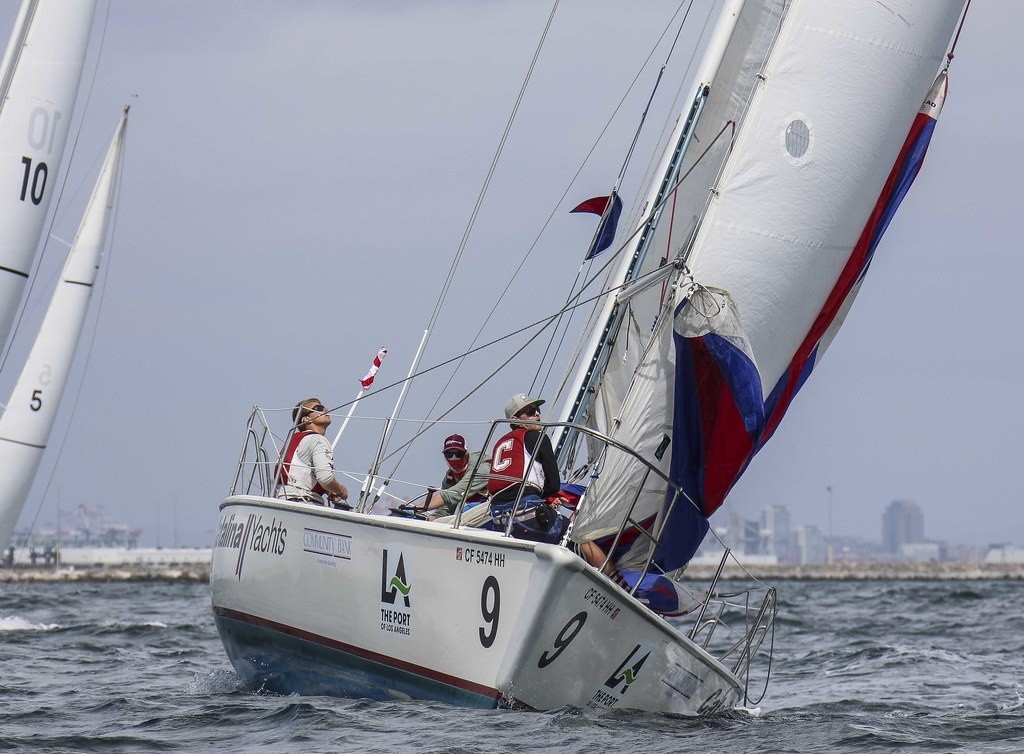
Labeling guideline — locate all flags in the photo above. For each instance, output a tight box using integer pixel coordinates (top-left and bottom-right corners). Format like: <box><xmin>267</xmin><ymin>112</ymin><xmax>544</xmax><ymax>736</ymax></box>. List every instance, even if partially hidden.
<box><xmin>357</xmin><ymin>347</ymin><xmax>387</xmax><ymax>390</ymax></box>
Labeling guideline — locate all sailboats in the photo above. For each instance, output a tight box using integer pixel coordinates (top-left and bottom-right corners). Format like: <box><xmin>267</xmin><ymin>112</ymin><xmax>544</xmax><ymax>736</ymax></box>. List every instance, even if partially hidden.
<box><xmin>211</xmin><ymin>0</ymin><xmax>972</xmax><ymax>713</ymax></box>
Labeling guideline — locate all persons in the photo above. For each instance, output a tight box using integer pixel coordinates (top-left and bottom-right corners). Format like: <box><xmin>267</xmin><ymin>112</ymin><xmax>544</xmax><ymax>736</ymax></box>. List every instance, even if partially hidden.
<box><xmin>274</xmin><ymin>398</ymin><xmax>347</xmax><ymax>506</ymax></box>
<box><xmin>488</xmin><ymin>392</ymin><xmax>636</xmax><ymax>597</ymax></box>
<box><xmin>403</xmin><ymin>433</ymin><xmax>492</xmax><ymax>530</ymax></box>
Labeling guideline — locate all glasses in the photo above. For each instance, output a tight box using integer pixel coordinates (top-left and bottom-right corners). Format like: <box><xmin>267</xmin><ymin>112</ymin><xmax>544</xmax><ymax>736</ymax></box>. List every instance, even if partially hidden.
<box><xmin>515</xmin><ymin>407</ymin><xmax>540</xmax><ymax>416</ymax></box>
<box><xmin>304</xmin><ymin>405</ymin><xmax>324</xmax><ymax>416</ymax></box>
<box><xmin>445</xmin><ymin>451</ymin><xmax>465</xmax><ymax>458</ymax></box>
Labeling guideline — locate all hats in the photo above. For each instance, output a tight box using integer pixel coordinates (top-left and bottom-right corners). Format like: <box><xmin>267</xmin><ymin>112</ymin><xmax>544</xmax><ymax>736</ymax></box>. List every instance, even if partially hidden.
<box><xmin>442</xmin><ymin>434</ymin><xmax>466</xmax><ymax>453</ymax></box>
<box><xmin>505</xmin><ymin>393</ymin><xmax>545</xmax><ymax>419</ymax></box>
